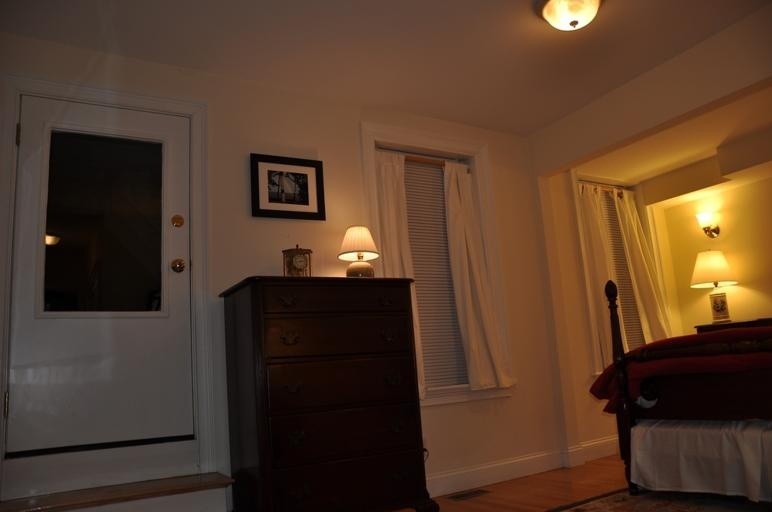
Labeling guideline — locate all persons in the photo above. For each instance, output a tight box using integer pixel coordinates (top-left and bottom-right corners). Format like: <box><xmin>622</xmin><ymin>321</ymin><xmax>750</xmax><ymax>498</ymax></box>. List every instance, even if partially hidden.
<box><xmin>280</xmin><ymin>189</ymin><xmax>286</xmax><ymax>202</ymax></box>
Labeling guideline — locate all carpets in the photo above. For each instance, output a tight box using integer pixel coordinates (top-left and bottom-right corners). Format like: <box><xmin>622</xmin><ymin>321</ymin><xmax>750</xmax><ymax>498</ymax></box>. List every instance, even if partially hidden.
<box><xmin>543</xmin><ymin>487</ymin><xmax>771</xmax><ymax>511</ymax></box>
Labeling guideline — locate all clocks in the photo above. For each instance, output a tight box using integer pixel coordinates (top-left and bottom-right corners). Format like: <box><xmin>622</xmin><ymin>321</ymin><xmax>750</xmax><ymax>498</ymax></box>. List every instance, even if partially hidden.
<box><xmin>282</xmin><ymin>244</ymin><xmax>313</xmax><ymax>278</ymax></box>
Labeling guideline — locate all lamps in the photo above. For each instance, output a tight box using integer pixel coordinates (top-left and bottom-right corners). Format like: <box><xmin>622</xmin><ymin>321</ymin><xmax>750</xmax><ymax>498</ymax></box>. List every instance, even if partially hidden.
<box><xmin>688</xmin><ymin>248</ymin><xmax>738</xmax><ymax>325</ymax></box>
<box><xmin>337</xmin><ymin>225</ymin><xmax>380</xmax><ymax>278</ymax></box>
<box><xmin>532</xmin><ymin>0</ymin><xmax>603</xmax><ymax>32</ymax></box>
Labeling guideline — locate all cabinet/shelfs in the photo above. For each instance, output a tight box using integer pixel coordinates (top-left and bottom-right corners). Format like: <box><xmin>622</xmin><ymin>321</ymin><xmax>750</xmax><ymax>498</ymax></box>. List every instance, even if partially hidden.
<box><xmin>219</xmin><ymin>275</ymin><xmax>441</xmax><ymax>512</ymax></box>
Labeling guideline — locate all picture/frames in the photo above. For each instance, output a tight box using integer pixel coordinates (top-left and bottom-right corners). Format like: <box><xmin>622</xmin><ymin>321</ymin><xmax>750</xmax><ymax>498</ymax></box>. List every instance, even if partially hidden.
<box><xmin>249</xmin><ymin>153</ymin><xmax>326</xmax><ymax>220</ymax></box>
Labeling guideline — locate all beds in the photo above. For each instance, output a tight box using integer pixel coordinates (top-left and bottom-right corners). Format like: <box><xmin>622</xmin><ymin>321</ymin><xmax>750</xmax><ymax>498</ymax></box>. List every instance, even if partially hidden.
<box><xmin>587</xmin><ymin>277</ymin><xmax>771</xmax><ymax>497</ymax></box>
